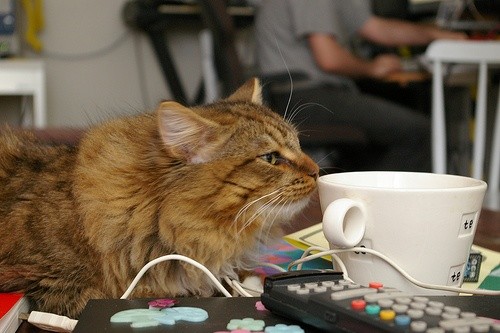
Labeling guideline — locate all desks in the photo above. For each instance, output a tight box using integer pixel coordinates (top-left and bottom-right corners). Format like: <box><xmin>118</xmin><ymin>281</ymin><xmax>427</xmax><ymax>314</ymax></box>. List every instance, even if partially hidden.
<box><xmin>0</xmin><ymin>56</ymin><xmax>47</xmax><ymax>131</ymax></box>
<box><xmin>278</xmin><ymin>174</ymin><xmax>500</xmax><ymax>265</ymax></box>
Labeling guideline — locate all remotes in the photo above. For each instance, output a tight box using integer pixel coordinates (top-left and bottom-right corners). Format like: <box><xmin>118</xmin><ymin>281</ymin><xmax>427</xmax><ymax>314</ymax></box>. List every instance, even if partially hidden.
<box><xmin>262</xmin><ymin>270</ymin><xmax>500</xmax><ymax>333</ymax></box>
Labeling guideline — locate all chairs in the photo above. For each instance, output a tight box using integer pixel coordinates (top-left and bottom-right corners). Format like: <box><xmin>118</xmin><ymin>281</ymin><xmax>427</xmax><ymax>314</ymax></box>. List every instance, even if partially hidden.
<box><xmin>200</xmin><ymin>0</ymin><xmax>363</xmax><ymax>145</ymax></box>
<box><xmin>425</xmin><ymin>40</ymin><xmax>500</xmax><ymax>213</ymax></box>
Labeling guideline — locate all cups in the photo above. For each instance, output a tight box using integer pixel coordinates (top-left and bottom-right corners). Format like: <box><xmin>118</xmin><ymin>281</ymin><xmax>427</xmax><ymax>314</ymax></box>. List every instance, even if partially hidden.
<box><xmin>317</xmin><ymin>171</ymin><xmax>487</xmax><ymax>297</ymax></box>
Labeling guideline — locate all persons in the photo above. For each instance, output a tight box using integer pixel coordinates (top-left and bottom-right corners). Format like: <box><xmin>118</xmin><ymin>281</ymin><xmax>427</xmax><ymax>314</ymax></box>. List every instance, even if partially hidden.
<box><xmin>253</xmin><ymin>0</ymin><xmax>470</xmax><ymax>173</ymax></box>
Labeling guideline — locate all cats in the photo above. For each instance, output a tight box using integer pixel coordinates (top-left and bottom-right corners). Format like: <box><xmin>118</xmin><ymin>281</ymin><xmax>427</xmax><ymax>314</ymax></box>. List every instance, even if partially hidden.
<box><xmin>0</xmin><ymin>76</ymin><xmax>320</xmax><ymax>320</ymax></box>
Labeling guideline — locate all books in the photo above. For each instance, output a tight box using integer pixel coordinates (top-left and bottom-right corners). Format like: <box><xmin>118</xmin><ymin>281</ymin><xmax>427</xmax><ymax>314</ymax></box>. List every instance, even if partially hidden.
<box><xmin>233</xmin><ymin>218</ymin><xmax>500</xmax><ymax>296</ymax></box>
<box><xmin>1</xmin><ymin>290</ymin><xmax>32</xmax><ymax>333</ymax></box>
<box><xmin>71</xmin><ymin>299</ymin><xmax>327</xmax><ymax>333</ymax></box>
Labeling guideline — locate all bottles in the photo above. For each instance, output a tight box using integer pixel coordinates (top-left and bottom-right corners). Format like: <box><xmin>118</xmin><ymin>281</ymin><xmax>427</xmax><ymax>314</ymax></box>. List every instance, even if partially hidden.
<box><xmin>0</xmin><ymin>0</ymin><xmax>16</xmax><ymax>57</ymax></box>
